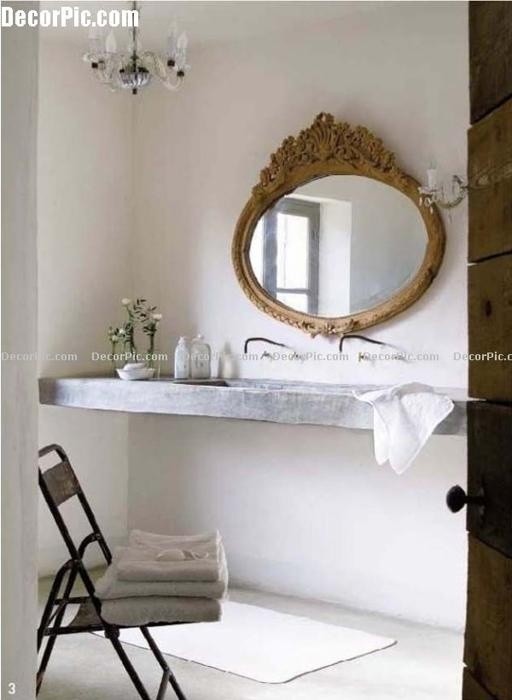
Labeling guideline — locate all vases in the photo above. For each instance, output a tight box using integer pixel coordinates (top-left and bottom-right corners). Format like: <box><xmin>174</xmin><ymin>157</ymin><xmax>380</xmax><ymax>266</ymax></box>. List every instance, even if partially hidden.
<box><xmin>104</xmin><ymin>350</ymin><xmax>162</xmax><ymax>380</ymax></box>
<box><xmin>125</xmin><ymin>356</ymin><xmax>138</xmax><ymax>364</ymax></box>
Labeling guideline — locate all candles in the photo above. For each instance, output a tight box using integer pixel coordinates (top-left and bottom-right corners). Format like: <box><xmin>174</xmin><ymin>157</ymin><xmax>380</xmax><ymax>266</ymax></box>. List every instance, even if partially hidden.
<box><xmin>426</xmin><ymin>161</ymin><xmax>438</xmax><ymax>188</ymax></box>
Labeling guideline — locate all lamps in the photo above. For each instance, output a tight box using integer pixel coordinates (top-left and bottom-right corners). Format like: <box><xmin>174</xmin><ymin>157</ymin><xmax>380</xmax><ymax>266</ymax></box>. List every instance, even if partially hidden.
<box><xmin>83</xmin><ymin>1</ymin><xmax>189</xmax><ymax>98</ymax></box>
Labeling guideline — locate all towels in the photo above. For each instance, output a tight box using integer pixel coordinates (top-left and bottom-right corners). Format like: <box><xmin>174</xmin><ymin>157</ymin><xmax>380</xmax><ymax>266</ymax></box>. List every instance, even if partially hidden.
<box><xmin>353</xmin><ymin>382</ymin><xmax>455</xmax><ymax>475</ymax></box>
<box><xmin>115</xmin><ymin>527</ymin><xmax>225</xmax><ymax>584</ymax></box>
<box><xmin>99</xmin><ymin>596</ymin><xmax>224</xmax><ymax>627</ymax></box>
<box><xmin>91</xmin><ymin>555</ymin><xmax>230</xmax><ymax>600</ymax></box>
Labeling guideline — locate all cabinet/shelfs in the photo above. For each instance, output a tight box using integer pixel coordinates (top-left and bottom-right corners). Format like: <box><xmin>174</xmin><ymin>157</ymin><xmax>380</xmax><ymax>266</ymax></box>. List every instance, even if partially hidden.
<box><xmin>461</xmin><ymin>669</ymin><xmax>490</xmax><ymax>699</ymax></box>
<box><xmin>467</xmin><ymin>255</ymin><xmax>511</xmax><ymax>407</ymax></box>
<box><xmin>459</xmin><ymin>102</ymin><xmax>512</xmax><ymax>262</ymax></box>
<box><xmin>465</xmin><ymin>537</ymin><xmax>510</xmax><ymax>699</ymax></box>
<box><xmin>464</xmin><ymin>404</ymin><xmax>511</xmax><ymax>558</ymax></box>
<box><xmin>468</xmin><ymin>1</ymin><xmax>511</xmax><ymax>122</ymax></box>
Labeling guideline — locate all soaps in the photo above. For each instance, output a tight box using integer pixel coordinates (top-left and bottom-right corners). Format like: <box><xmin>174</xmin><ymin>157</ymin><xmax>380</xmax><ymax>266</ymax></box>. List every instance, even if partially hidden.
<box><xmin>123</xmin><ymin>363</ymin><xmax>146</xmax><ymax>371</ymax></box>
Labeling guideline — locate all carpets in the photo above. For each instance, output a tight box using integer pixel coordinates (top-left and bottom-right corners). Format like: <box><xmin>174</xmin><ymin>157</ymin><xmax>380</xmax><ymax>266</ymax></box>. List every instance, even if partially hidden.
<box><xmin>87</xmin><ymin>591</ymin><xmax>396</xmax><ymax>684</ymax></box>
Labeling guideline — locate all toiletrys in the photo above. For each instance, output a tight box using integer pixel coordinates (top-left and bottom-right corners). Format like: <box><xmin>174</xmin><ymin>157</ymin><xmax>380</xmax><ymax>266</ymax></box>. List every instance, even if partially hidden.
<box><xmin>174</xmin><ymin>336</ymin><xmax>189</xmax><ymax>379</ymax></box>
<box><xmin>190</xmin><ymin>334</ymin><xmax>212</xmax><ymax>378</ymax></box>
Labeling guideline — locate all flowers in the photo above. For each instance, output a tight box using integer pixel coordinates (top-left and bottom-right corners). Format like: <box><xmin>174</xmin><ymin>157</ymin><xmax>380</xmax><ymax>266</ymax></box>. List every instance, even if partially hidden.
<box><xmin>105</xmin><ymin>295</ymin><xmax>163</xmax><ymax>364</ymax></box>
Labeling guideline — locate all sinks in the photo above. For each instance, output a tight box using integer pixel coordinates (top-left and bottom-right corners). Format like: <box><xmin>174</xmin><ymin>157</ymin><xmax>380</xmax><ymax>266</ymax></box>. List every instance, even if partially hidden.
<box><xmin>173</xmin><ymin>381</ymin><xmax>352</xmax><ymax>394</ymax></box>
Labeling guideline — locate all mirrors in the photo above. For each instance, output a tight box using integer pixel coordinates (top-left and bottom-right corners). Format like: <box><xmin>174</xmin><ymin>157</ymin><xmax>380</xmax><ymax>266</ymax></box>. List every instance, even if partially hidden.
<box><xmin>229</xmin><ymin>109</ymin><xmax>447</xmax><ymax>344</ymax></box>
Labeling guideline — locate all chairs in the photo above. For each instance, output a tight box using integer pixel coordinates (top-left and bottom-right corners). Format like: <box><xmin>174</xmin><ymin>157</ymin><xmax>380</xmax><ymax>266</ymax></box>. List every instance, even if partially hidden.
<box><xmin>33</xmin><ymin>443</ymin><xmax>187</xmax><ymax>700</ymax></box>
